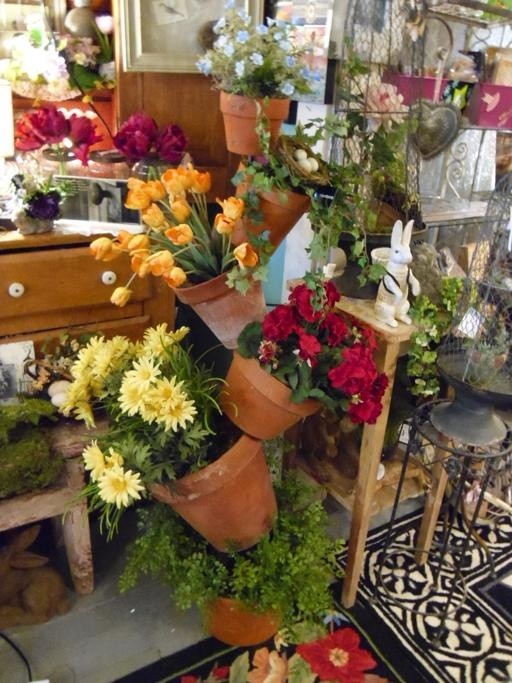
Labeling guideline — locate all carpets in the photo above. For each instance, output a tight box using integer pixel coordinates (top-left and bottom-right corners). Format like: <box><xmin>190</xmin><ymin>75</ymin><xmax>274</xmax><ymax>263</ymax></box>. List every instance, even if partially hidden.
<box><xmin>108</xmin><ymin>492</ymin><xmax>512</xmax><ymax>683</ymax></box>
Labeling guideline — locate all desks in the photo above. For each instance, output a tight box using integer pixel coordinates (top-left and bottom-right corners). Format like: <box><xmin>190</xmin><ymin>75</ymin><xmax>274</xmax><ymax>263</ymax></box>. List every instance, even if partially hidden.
<box><xmin>282</xmin><ymin>277</ymin><xmax>497</xmax><ymax>610</ymax></box>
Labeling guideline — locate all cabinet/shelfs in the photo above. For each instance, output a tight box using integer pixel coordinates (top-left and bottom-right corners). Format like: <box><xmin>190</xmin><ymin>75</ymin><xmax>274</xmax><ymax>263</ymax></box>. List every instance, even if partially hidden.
<box><xmin>311</xmin><ymin>1</ymin><xmax>512</xmax><ymax>305</ymax></box>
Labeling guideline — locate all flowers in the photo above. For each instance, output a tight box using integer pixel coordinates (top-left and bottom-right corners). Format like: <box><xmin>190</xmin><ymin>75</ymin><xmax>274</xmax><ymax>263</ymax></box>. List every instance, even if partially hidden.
<box><xmin>0</xmin><ymin>12</ymin><xmax>383</xmax><ymax>683</ymax></box>
